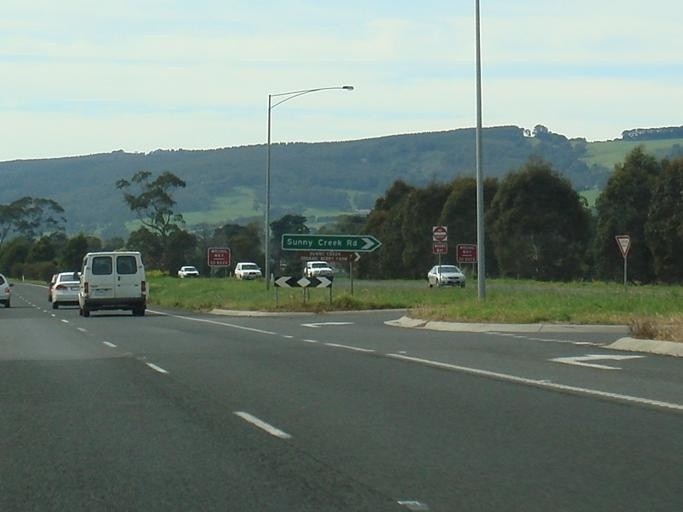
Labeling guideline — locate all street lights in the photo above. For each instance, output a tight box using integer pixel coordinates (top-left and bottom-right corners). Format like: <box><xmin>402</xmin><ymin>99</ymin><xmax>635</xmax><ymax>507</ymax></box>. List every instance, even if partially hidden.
<box><xmin>263</xmin><ymin>85</ymin><xmax>354</xmax><ymax>293</ymax></box>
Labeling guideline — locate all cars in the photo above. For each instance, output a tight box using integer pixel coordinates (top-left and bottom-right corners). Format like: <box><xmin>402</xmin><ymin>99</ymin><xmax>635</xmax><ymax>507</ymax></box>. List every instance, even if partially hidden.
<box><xmin>0</xmin><ymin>273</ymin><xmax>16</xmax><ymax>307</ymax></box>
<box><xmin>426</xmin><ymin>264</ymin><xmax>465</xmax><ymax>287</ymax></box>
<box><xmin>46</xmin><ymin>270</ymin><xmax>84</xmax><ymax>309</ymax></box>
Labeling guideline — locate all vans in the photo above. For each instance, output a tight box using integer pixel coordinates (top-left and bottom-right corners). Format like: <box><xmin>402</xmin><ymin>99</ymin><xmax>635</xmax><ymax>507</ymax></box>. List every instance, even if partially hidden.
<box><xmin>71</xmin><ymin>250</ymin><xmax>149</xmax><ymax>319</ymax></box>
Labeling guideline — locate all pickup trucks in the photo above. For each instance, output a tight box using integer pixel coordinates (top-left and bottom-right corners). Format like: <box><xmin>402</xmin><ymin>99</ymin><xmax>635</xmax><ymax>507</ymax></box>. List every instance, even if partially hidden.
<box><xmin>177</xmin><ymin>265</ymin><xmax>200</xmax><ymax>278</ymax></box>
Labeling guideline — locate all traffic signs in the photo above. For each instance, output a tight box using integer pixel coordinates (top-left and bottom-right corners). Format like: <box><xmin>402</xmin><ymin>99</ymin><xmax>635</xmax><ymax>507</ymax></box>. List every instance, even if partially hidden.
<box><xmin>281</xmin><ymin>232</ymin><xmax>383</xmax><ymax>252</ymax></box>
<box><xmin>294</xmin><ymin>249</ymin><xmax>360</xmax><ymax>263</ymax></box>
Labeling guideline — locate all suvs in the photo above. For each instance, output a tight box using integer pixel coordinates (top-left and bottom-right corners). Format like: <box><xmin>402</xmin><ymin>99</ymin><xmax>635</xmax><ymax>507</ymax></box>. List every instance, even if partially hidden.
<box><xmin>234</xmin><ymin>262</ymin><xmax>262</xmax><ymax>279</ymax></box>
<box><xmin>302</xmin><ymin>260</ymin><xmax>334</xmax><ymax>279</ymax></box>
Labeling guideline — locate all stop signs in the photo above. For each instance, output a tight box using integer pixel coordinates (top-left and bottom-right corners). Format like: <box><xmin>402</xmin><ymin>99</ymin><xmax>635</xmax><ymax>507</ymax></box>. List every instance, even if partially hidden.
<box><xmin>432</xmin><ymin>226</ymin><xmax>448</xmax><ymax>242</ymax></box>
